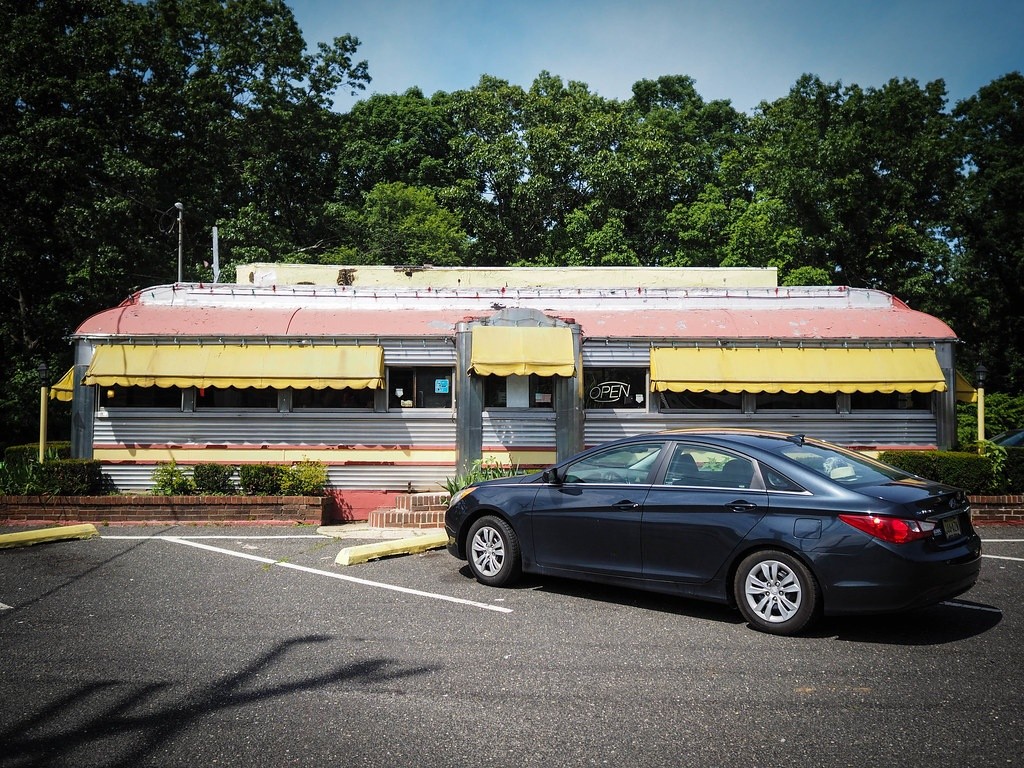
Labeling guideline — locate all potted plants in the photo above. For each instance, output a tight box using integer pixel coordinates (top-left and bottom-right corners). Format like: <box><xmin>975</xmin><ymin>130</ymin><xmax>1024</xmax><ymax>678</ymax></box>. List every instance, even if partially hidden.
<box><xmin>1</xmin><ymin>445</ymin><xmax>1022</xmax><ymax>524</ymax></box>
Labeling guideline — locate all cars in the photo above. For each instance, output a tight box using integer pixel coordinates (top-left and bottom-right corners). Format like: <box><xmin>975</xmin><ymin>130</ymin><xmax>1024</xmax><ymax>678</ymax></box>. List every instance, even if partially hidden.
<box><xmin>443</xmin><ymin>430</ymin><xmax>983</xmax><ymax>636</ymax></box>
